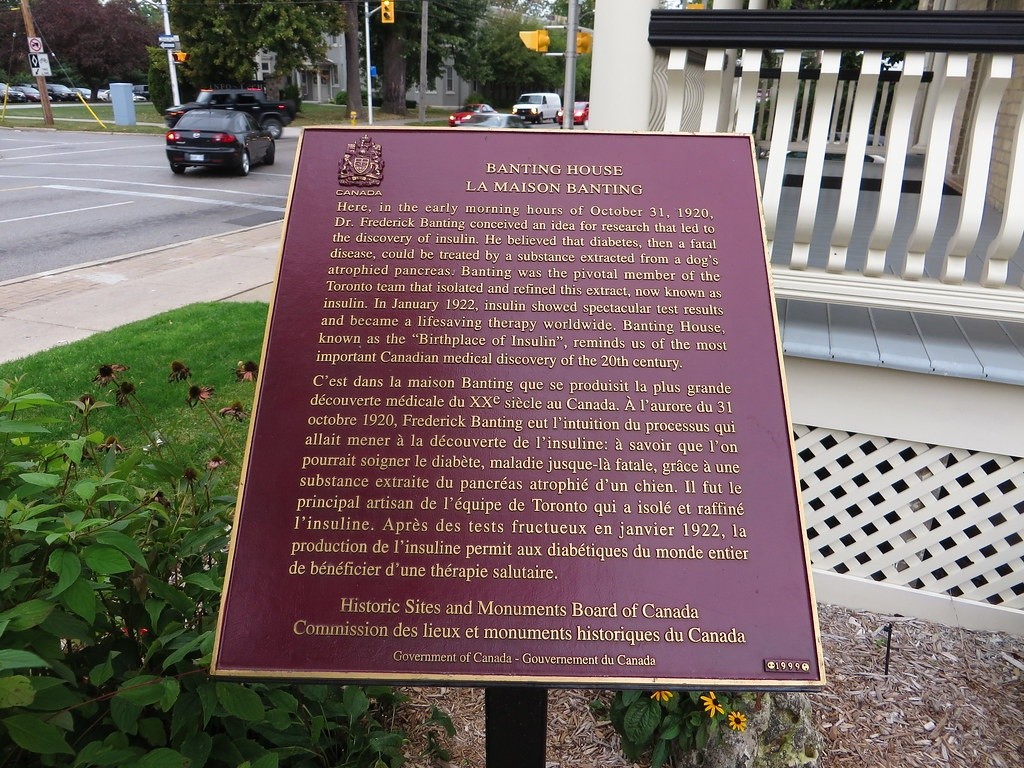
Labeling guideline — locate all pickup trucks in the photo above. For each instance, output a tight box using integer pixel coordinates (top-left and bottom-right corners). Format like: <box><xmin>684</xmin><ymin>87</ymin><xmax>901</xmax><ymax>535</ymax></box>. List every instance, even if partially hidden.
<box><xmin>164</xmin><ymin>89</ymin><xmax>293</xmax><ymax>141</ymax></box>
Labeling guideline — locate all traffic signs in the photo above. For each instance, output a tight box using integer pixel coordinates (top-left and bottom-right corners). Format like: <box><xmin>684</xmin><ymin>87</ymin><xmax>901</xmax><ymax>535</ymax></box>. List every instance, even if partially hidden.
<box><xmin>158</xmin><ymin>36</ymin><xmax>175</xmax><ymax>49</ymax></box>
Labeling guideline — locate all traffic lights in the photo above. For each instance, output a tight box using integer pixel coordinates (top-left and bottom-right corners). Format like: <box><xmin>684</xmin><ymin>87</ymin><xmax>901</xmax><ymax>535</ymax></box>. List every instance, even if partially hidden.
<box><xmin>383</xmin><ymin>1</ymin><xmax>391</xmax><ymax>20</ymax></box>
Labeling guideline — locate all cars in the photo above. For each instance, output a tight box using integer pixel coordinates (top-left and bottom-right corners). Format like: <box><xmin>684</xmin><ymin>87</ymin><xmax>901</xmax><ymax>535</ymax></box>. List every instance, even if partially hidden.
<box><xmin>557</xmin><ymin>101</ymin><xmax>589</xmax><ymax>125</ymax></box>
<box><xmin>447</xmin><ymin>103</ymin><xmax>498</xmax><ymax>127</ymax></box>
<box><xmin>0</xmin><ymin>83</ymin><xmax>147</xmax><ymax>104</ymax></box>
<box><xmin>165</xmin><ymin>107</ymin><xmax>276</xmax><ymax>176</ymax></box>
<box><xmin>459</xmin><ymin>113</ymin><xmax>532</xmax><ymax>129</ymax></box>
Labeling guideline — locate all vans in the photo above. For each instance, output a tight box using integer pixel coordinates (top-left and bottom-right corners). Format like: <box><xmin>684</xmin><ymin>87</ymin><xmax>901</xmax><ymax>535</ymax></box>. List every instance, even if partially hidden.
<box><xmin>511</xmin><ymin>92</ymin><xmax>562</xmax><ymax>124</ymax></box>
